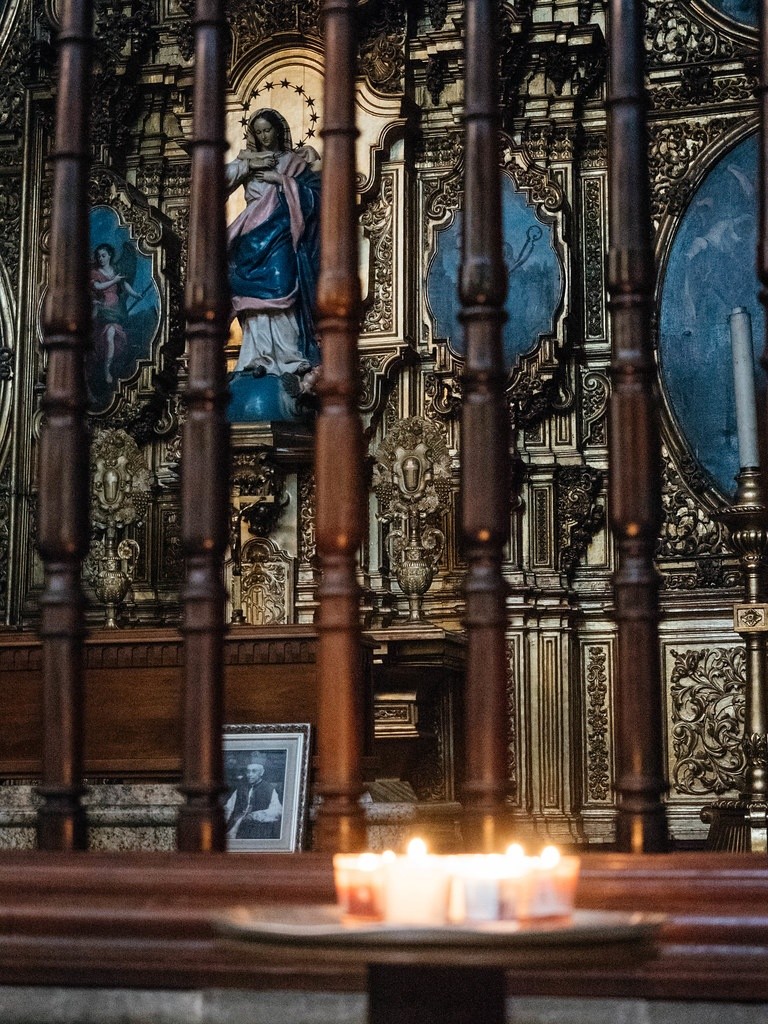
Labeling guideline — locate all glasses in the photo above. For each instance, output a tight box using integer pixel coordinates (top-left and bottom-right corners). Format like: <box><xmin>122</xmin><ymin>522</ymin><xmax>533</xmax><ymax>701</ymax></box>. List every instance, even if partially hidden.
<box><xmin>246</xmin><ymin>769</ymin><xmax>262</xmax><ymax>774</ymax></box>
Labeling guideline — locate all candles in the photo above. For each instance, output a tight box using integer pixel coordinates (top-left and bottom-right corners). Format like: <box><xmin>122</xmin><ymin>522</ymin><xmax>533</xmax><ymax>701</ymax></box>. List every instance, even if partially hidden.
<box><xmin>332</xmin><ymin>841</ymin><xmax>577</xmax><ymax>923</ymax></box>
<box><xmin>732</xmin><ymin>312</ymin><xmax>761</xmax><ymax>469</ymax></box>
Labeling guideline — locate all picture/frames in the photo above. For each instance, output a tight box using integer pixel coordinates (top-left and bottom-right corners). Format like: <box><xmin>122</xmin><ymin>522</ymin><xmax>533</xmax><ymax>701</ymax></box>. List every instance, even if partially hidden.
<box><xmin>220</xmin><ymin>721</ymin><xmax>314</xmax><ymax>853</ymax></box>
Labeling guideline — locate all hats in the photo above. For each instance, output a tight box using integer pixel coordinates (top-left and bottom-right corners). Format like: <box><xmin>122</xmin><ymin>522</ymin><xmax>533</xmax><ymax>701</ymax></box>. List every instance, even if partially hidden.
<box><xmin>246</xmin><ymin>752</ymin><xmax>265</xmax><ymax>766</ymax></box>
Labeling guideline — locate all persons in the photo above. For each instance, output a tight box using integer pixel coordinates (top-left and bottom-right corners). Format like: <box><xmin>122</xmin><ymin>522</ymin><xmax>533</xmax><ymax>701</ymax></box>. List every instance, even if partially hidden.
<box><xmin>224</xmin><ymin>751</ymin><xmax>284</xmax><ymax>840</ymax></box>
<box><xmin>220</xmin><ymin>108</ymin><xmax>324</xmax><ymax>373</ymax></box>
